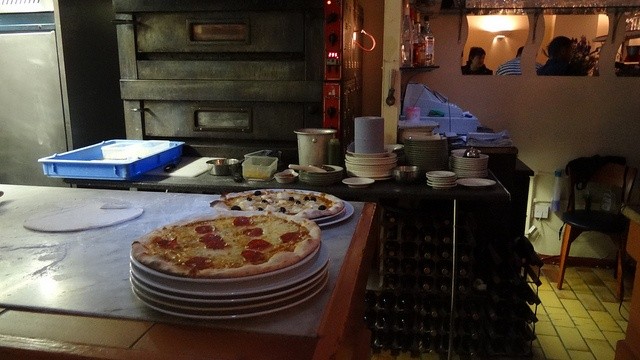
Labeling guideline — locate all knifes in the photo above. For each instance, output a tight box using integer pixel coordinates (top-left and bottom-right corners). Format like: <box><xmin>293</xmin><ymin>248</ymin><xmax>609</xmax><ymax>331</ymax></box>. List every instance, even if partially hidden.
<box><xmin>164</xmin><ymin>159</ymin><xmax>181</xmax><ymax>173</ymax></box>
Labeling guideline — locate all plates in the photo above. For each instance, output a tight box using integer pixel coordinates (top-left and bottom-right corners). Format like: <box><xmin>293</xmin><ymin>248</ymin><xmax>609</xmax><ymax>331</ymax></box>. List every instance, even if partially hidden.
<box><xmin>344</xmin><ymin>147</ymin><xmax>399</xmax><ymax>177</ymax></box>
<box><xmin>376</xmin><ymin>178</ymin><xmax>392</xmax><ymax>181</ymax></box>
<box><xmin>298</xmin><ymin>164</ymin><xmax>344</xmax><ymax>185</ymax></box>
<box><xmin>310</xmin><ymin>201</ymin><xmax>347</xmax><ymax>223</ymax></box>
<box><xmin>342</xmin><ymin>177</ymin><xmax>375</xmax><ymax>189</ymax></box>
<box><xmin>426</xmin><ymin>171</ymin><xmax>457</xmax><ymax>190</ymax></box>
<box><xmin>129</xmin><ymin>241</ymin><xmax>331</xmax><ymax>323</ymax></box>
<box><xmin>458</xmin><ymin>176</ymin><xmax>495</xmax><ymax>188</ymax></box>
<box><xmin>404</xmin><ymin>137</ymin><xmax>448</xmax><ymax>165</ymax></box>
<box><xmin>421</xmin><ymin>165</ymin><xmax>449</xmax><ymax>171</ymax></box>
<box><xmin>449</xmin><ymin>147</ymin><xmax>489</xmax><ymax>178</ymax></box>
<box><xmin>318</xmin><ymin>202</ymin><xmax>355</xmax><ymax>228</ymax></box>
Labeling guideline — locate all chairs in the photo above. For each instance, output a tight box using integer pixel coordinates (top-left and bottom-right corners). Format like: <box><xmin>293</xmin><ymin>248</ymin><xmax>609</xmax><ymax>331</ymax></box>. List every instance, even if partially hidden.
<box><xmin>556</xmin><ymin>153</ymin><xmax>631</xmax><ymax>302</ymax></box>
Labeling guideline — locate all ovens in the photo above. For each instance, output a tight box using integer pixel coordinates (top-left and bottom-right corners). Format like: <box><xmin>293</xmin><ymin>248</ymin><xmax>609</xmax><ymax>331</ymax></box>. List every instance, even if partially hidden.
<box><xmin>113</xmin><ymin>1</ymin><xmax>377</xmax><ymax>158</ymax></box>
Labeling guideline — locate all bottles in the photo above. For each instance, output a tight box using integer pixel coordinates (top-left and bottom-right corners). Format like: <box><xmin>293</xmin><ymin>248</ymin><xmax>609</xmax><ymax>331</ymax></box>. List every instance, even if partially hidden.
<box><xmin>384</xmin><ymin>212</ymin><xmax>396</xmax><ymax>224</ymax></box>
<box><xmin>441</xmin><ymin>214</ymin><xmax>451</xmax><ymax>227</ymax></box>
<box><xmin>418</xmin><ymin>261</ymin><xmax>436</xmax><ymax>275</ymax></box>
<box><xmin>414</xmin><ymin>218</ymin><xmax>426</xmax><ymax>230</ymax></box>
<box><xmin>439</xmin><ymin>246</ymin><xmax>451</xmax><ymax>259</ymax></box>
<box><xmin>455</xmin><ymin>201</ymin><xmax>506</xmax><ymax>359</ymax></box>
<box><xmin>520</xmin><ymin>260</ymin><xmax>541</xmax><ymax>286</ymax></box>
<box><xmin>419</xmin><ymin>243</ymin><xmax>436</xmax><ymax>261</ymax></box>
<box><xmin>440</xmin><ymin>265</ymin><xmax>452</xmax><ymax>278</ymax></box>
<box><xmin>419</xmin><ymin>228</ymin><xmax>434</xmax><ymax>242</ymax></box>
<box><xmin>440</xmin><ymin>231</ymin><xmax>453</xmax><ymax>243</ymax></box>
<box><xmin>362</xmin><ymin>288</ymin><xmax>452</xmax><ymax>360</ymax></box>
<box><xmin>437</xmin><ymin>279</ymin><xmax>451</xmax><ymax>292</ymax></box>
<box><xmin>419</xmin><ymin>278</ymin><xmax>434</xmax><ymax>291</ymax></box>
<box><xmin>327</xmin><ymin>133</ymin><xmax>342</xmax><ymax>166</ymax></box>
<box><xmin>511</xmin><ymin>235</ymin><xmax>542</xmax><ymax>267</ymax></box>
<box><xmin>399</xmin><ymin>0</ymin><xmax>412</xmax><ymax>68</ymax></box>
<box><xmin>412</xmin><ymin>9</ymin><xmax>426</xmax><ymax>67</ymax></box>
<box><xmin>384</xmin><ymin>241</ymin><xmax>398</xmax><ymax>256</ymax></box>
<box><xmin>421</xmin><ymin>15</ymin><xmax>435</xmax><ymax>65</ymax></box>
<box><xmin>506</xmin><ymin>283</ymin><xmax>541</xmax><ymax>359</ymax></box>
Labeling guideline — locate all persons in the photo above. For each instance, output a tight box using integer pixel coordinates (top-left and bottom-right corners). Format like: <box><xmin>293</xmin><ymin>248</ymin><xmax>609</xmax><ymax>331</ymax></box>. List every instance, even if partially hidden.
<box><xmin>538</xmin><ymin>36</ymin><xmax>572</xmax><ymax>77</ymax></box>
<box><xmin>496</xmin><ymin>47</ymin><xmax>545</xmax><ymax>76</ymax></box>
<box><xmin>463</xmin><ymin>46</ymin><xmax>494</xmax><ymax>76</ymax></box>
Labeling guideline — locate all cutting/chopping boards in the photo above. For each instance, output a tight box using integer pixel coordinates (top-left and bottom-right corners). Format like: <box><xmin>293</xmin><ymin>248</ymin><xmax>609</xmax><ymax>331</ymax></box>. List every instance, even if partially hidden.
<box><xmin>142</xmin><ymin>158</ymin><xmax>227</xmax><ymax>178</ymax></box>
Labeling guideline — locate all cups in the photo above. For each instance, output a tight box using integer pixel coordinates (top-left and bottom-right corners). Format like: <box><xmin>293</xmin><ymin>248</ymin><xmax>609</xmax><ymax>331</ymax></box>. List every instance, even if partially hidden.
<box><xmin>405</xmin><ymin>106</ymin><xmax>420</xmax><ymax>126</ymax></box>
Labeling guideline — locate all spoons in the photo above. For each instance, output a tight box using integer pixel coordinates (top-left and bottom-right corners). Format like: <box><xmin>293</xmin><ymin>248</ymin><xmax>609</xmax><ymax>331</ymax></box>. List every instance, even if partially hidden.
<box><xmin>386</xmin><ymin>69</ymin><xmax>397</xmax><ymax>106</ymax></box>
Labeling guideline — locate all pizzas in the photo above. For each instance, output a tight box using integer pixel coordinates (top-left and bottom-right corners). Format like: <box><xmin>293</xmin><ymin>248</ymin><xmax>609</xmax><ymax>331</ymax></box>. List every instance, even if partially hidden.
<box><xmin>222</xmin><ymin>191</ymin><xmax>344</xmax><ymax>221</ymax></box>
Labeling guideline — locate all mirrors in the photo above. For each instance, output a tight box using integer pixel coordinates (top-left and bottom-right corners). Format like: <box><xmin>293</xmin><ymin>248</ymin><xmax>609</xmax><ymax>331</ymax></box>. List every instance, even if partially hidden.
<box><xmin>614</xmin><ymin>10</ymin><xmax>640</xmax><ymax>77</ymax></box>
<box><xmin>536</xmin><ymin>15</ymin><xmax>609</xmax><ymax>77</ymax></box>
<box><xmin>460</xmin><ymin>15</ymin><xmax>530</xmax><ymax>76</ymax></box>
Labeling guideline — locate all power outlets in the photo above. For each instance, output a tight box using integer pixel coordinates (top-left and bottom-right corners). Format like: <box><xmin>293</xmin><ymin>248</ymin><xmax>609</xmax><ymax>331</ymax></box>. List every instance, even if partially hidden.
<box><xmin>534</xmin><ymin>204</ymin><xmax>549</xmax><ymax>218</ymax></box>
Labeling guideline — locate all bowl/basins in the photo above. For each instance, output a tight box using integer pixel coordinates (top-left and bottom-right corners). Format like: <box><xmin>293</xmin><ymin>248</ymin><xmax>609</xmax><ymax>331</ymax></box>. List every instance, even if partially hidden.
<box><xmin>207</xmin><ymin>157</ymin><xmax>244</xmax><ymax>176</ymax></box>
<box><xmin>391</xmin><ymin>166</ymin><xmax>422</xmax><ymax>187</ymax></box>
<box><xmin>274</xmin><ymin>168</ymin><xmax>298</xmax><ymax>184</ymax></box>
